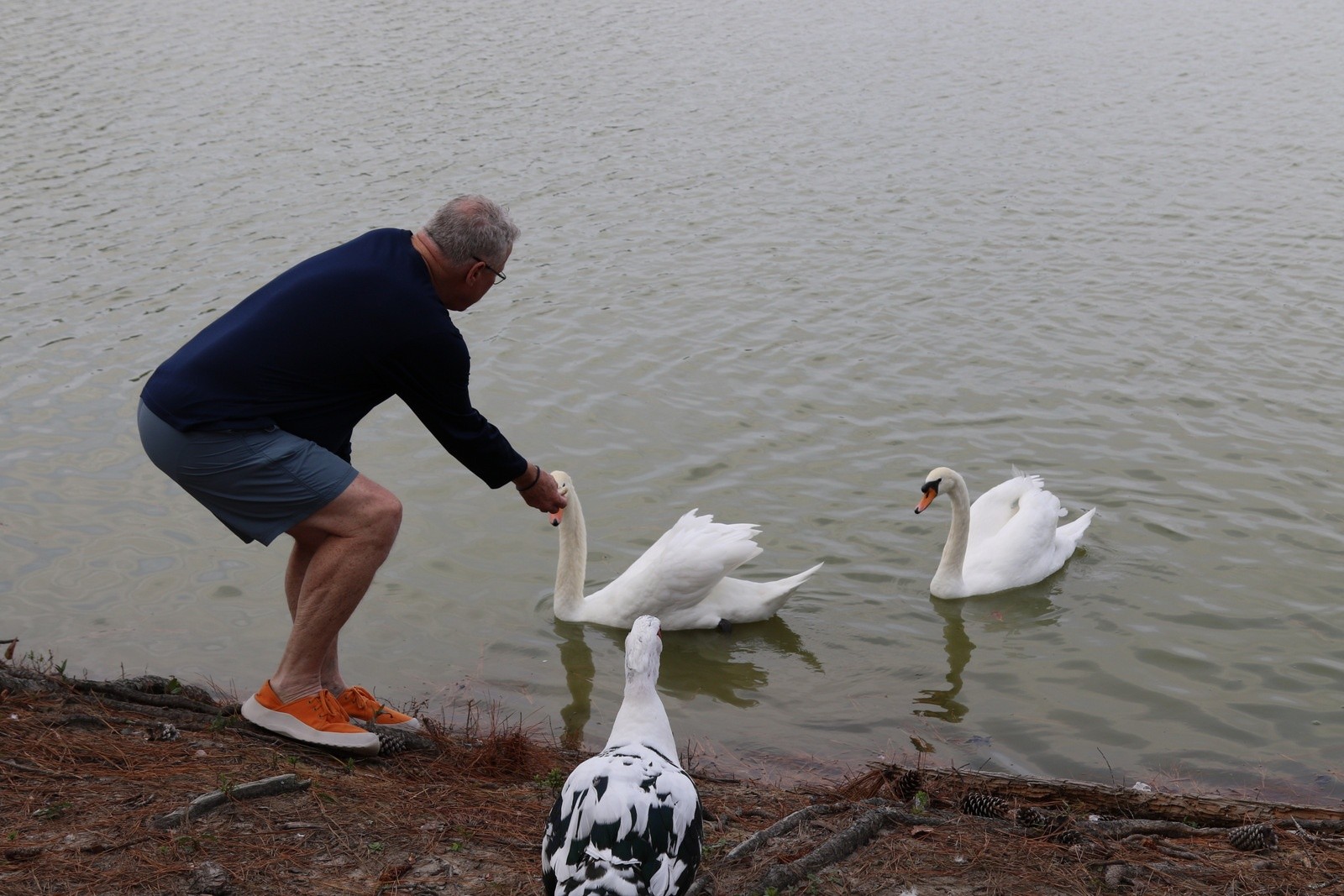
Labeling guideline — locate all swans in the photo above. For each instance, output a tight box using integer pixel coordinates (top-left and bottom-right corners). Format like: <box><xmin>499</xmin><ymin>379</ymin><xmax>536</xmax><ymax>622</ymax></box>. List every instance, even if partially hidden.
<box><xmin>549</xmin><ymin>470</ymin><xmax>824</xmax><ymax>631</ymax></box>
<box><xmin>914</xmin><ymin>461</ymin><xmax>1096</xmax><ymax>598</ymax></box>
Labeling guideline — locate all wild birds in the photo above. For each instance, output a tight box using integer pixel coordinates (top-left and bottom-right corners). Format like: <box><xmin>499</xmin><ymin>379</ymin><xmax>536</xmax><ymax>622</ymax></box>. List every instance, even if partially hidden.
<box><xmin>535</xmin><ymin>616</ymin><xmax>705</xmax><ymax>896</ymax></box>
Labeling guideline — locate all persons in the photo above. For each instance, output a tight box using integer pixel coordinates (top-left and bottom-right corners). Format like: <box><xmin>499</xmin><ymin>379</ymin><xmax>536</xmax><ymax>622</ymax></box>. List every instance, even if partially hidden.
<box><xmin>136</xmin><ymin>193</ymin><xmax>569</xmax><ymax>752</ymax></box>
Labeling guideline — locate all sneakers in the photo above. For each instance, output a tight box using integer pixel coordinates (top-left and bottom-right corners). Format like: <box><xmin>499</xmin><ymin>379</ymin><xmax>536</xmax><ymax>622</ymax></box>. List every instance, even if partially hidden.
<box><xmin>335</xmin><ymin>685</ymin><xmax>420</xmax><ymax>733</ymax></box>
<box><xmin>241</xmin><ymin>678</ymin><xmax>380</xmax><ymax>755</ymax></box>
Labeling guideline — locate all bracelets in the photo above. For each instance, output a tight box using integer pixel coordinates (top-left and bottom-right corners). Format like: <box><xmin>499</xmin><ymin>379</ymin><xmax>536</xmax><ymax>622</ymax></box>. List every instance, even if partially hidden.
<box><xmin>516</xmin><ymin>465</ymin><xmax>541</xmax><ymax>491</ymax></box>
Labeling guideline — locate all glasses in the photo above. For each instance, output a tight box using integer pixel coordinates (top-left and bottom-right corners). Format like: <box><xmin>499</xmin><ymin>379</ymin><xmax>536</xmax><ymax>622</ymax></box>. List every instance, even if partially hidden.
<box><xmin>472</xmin><ymin>255</ymin><xmax>506</xmax><ymax>285</ymax></box>
